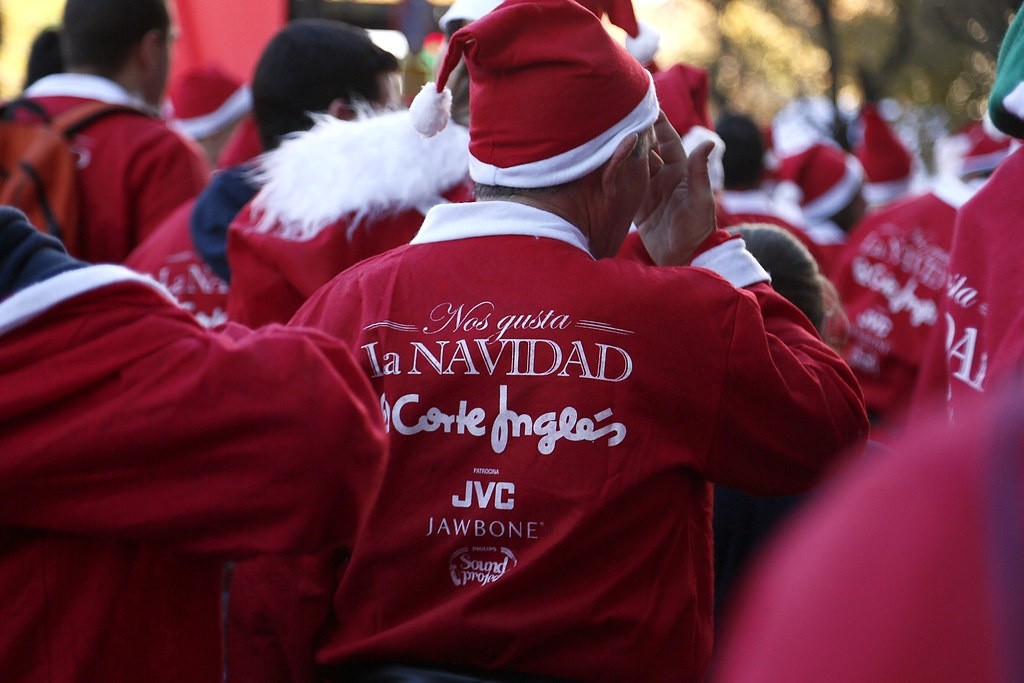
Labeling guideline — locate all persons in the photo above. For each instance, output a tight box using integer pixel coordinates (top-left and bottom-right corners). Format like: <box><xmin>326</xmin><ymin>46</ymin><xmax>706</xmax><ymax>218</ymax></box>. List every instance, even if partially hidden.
<box><xmin>645</xmin><ymin>5</ymin><xmax>1024</xmax><ymax>682</ymax></box>
<box><xmin>166</xmin><ymin>59</ymin><xmax>254</xmax><ymax>174</ymax></box>
<box><xmin>271</xmin><ymin>0</ymin><xmax>870</xmax><ymax>682</ymax></box>
<box><xmin>1</xmin><ymin>202</ymin><xmax>386</xmax><ymax>681</ymax></box>
<box><xmin>122</xmin><ymin>19</ymin><xmax>406</xmax><ymax>326</ymax></box>
<box><xmin>0</xmin><ymin>0</ymin><xmax>209</xmax><ymax>266</ymax></box>
<box><xmin>713</xmin><ymin>220</ymin><xmax>859</xmax><ymax>634</ymax></box>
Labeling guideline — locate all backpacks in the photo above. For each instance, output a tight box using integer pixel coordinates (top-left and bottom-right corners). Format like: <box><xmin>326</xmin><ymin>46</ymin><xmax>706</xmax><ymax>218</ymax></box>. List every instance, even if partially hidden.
<box><xmin>0</xmin><ymin>96</ymin><xmax>136</xmax><ymax>262</ymax></box>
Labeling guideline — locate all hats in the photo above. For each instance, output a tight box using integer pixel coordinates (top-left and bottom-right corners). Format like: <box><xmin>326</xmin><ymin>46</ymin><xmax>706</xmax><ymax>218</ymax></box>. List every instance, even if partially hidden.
<box><xmin>439</xmin><ymin>0</ymin><xmax>659</xmax><ymax>68</ymax></box>
<box><xmin>408</xmin><ymin>0</ymin><xmax>659</xmax><ymax>189</ymax></box>
<box><xmin>767</xmin><ymin>136</ymin><xmax>863</xmax><ymax>231</ymax></box>
<box><xmin>849</xmin><ymin>103</ymin><xmax>916</xmax><ymax>204</ymax></box>
<box><xmin>982</xmin><ymin>2</ymin><xmax>1024</xmax><ymax>144</ymax></box>
<box><xmin>653</xmin><ymin>62</ymin><xmax>726</xmax><ymax>192</ymax></box>
